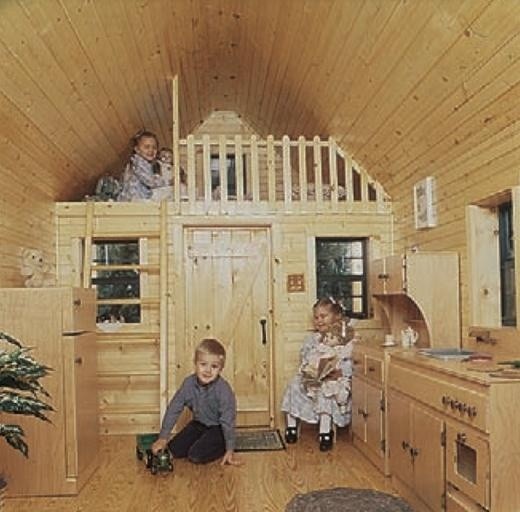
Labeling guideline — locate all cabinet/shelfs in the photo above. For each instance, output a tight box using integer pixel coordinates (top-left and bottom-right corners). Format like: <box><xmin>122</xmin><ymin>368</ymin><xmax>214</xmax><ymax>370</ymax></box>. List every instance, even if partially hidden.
<box><xmin>1</xmin><ymin>290</ymin><xmax>100</xmax><ymax>497</ymax></box>
<box><xmin>347</xmin><ymin>343</ymin><xmax>391</xmax><ymax>477</ymax></box>
<box><xmin>371</xmin><ymin>253</ymin><xmax>406</xmax><ymax>296</ymax></box>
<box><xmin>387</xmin><ymin>393</ymin><xmax>445</xmax><ymax>512</ymax></box>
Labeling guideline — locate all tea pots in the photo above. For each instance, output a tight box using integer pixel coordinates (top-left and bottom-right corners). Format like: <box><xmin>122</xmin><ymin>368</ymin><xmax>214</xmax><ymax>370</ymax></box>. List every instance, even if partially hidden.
<box><xmin>400</xmin><ymin>325</ymin><xmax>419</xmax><ymax>347</ymax></box>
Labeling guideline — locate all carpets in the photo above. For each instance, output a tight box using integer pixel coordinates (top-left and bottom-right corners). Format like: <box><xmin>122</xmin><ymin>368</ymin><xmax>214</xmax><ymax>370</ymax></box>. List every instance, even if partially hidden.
<box><xmin>285</xmin><ymin>488</ymin><xmax>414</xmax><ymax>512</ymax></box>
<box><xmin>223</xmin><ymin>429</ymin><xmax>287</xmax><ymax>452</ymax></box>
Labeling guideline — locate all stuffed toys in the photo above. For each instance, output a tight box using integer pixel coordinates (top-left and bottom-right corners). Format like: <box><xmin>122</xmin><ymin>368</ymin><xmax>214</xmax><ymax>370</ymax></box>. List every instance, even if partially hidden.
<box><xmin>19</xmin><ymin>248</ymin><xmax>55</xmax><ymax>288</ymax></box>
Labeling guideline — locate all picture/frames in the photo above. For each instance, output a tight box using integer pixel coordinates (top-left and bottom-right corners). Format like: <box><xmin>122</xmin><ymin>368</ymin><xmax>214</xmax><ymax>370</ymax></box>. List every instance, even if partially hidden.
<box><xmin>413</xmin><ymin>178</ymin><xmax>436</xmax><ymax>228</ymax></box>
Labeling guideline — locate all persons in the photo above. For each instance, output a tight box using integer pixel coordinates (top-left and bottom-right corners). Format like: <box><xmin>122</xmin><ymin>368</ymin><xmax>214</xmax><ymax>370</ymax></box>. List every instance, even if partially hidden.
<box><xmin>279</xmin><ymin>293</ymin><xmax>356</xmax><ymax>452</ymax></box>
<box><xmin>116</xmin><ymin>129</ymin><xmax>185</xmax><ymax>203</ymax></box>
<box><xmin>151</xmin><ymin>338</ymin><xmax>246</xmax><ymax>467</ymax></box>
<box><xmin>301</xmin><ymin>318</ymin><xmax>354</xmax><ymax>394</ymax></box>
<box><xmin>154</xmin><ymin>147</ymin><xmax>174</xmax><ymax>181</ymax></box>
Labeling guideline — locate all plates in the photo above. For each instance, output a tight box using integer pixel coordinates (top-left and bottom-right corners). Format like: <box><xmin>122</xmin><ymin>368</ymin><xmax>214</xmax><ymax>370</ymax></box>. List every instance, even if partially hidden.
<box><xmin>381</xmin><ymin>342</ymin><xmax>395</xmax><ymax>347</ymax></box>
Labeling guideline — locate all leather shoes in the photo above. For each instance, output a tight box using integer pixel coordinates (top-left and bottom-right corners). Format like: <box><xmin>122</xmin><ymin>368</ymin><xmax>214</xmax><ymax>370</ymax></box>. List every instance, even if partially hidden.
<box><xmin>319</xmin><ymin>430</ymin><xmax>334</xmax><ymax>451</ymax></box>
<box><xmin>285</xmin><ymin>427</ymin><xmax>297</xmax><ymax>444</ymax></box>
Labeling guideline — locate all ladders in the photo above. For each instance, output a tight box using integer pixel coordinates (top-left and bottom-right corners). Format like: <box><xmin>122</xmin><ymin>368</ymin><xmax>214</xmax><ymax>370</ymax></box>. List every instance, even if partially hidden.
<box><xmin>81</xmin><ymin>199</ymin><xmax>169</xmax><ymax>432</ymax></box>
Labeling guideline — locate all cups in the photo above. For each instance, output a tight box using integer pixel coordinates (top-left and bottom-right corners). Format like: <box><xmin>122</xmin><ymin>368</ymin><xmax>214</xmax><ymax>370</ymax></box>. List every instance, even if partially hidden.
<box><xmin>384</xmin><ymin>333</ymin><xmax>394</xmax><ymax>344</ymax></box>
